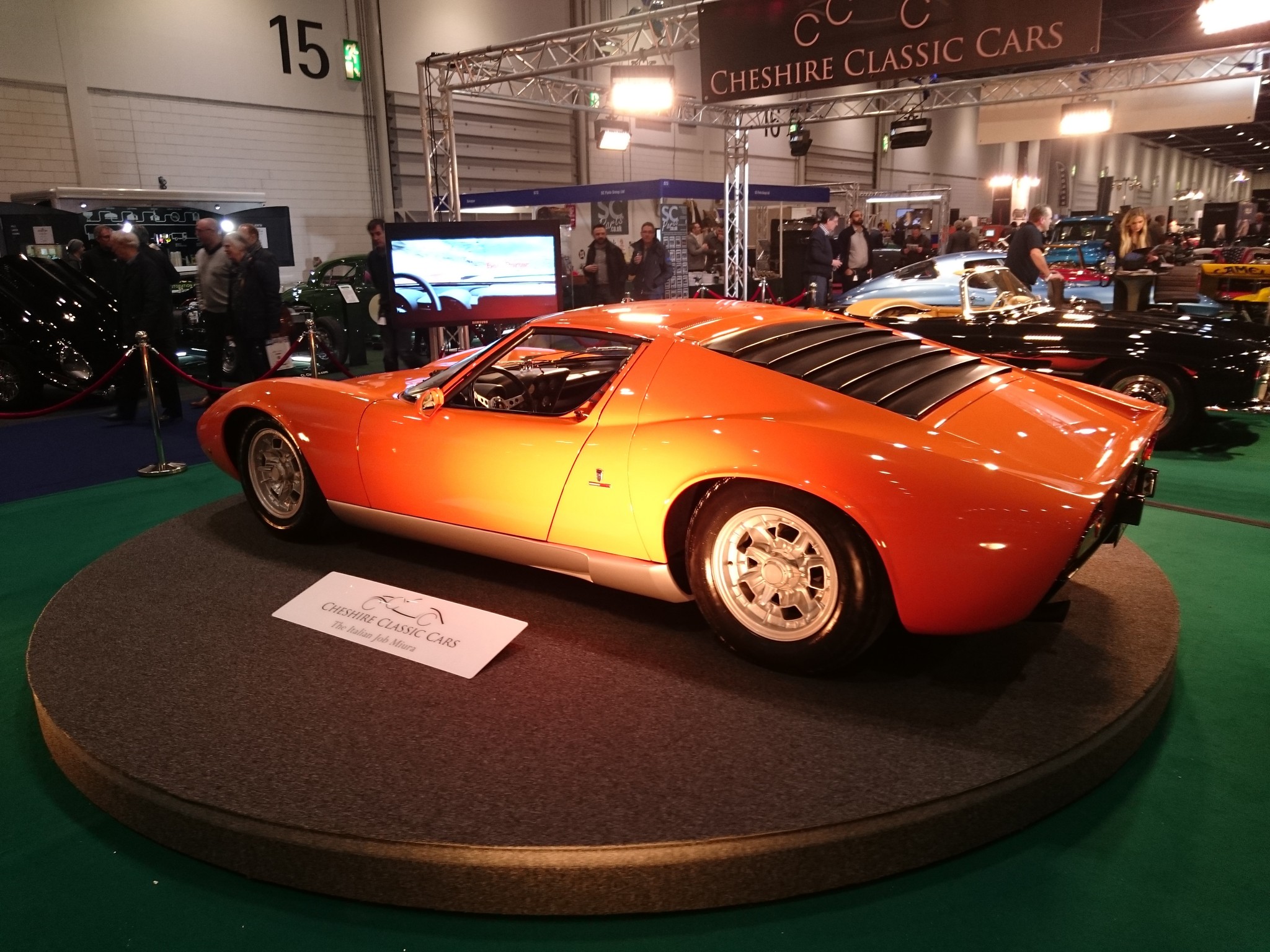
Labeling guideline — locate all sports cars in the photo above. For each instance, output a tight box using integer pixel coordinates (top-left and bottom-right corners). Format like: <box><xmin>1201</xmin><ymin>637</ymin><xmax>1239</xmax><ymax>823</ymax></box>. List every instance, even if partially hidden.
<box><xmin>904</xmin><ymin>266</ymin><xmax>1270</xmax><ymax>447</ymax></box>
<box><xmin>192</xmin><ymin>297</ymin><xmax>1170</xmax><ymax>681</ymax></box>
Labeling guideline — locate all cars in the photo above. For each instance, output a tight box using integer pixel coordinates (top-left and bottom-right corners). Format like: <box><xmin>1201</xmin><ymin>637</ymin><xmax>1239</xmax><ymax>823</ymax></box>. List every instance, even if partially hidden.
<box><xmin>277</xmin><ymin>254</ymin><xmax>503</xmax><ymax>374</ymax></box>
<box><xmin>828</xmin><ymin>212</ymin><xmax>1270</xmax><ymax>318</ymax></box>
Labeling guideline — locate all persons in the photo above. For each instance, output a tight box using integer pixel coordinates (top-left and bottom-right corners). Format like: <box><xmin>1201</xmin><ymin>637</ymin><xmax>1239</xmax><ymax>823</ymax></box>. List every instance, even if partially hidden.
<box><xmin>808</xmin><ymin>203</ymin><xmax>1267</xmax><ymax>307</ymax></box>
<box><xmin>64</xmin><ymin>218</ymin><xmax>283</xmax><ymax>424</ymax></box>
<box><xmin>366</xmin><ymin>218</ymin><xmax>425</xmax><ymax>373</ymax></box>
<box><xmin>582</xmin><ymin>223</ymin><xmax>628</xmax><ymax>307</ymax></box>
<box><xmin>627</xmin><ymin>221</ymin><xmax>673</xmax><ymax>301</ymax></box>
<box><xmin>686</xmin><ymin>222</ymin><xmax>724</xmax><ymax>272</ymax></box>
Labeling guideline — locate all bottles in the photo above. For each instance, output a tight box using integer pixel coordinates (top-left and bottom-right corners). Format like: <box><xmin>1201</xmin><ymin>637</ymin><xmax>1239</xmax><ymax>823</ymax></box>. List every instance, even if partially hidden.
<box><xmin>1104</xmin><ymin>251</ymin><xmax>1116</xmax><ymax>275</ymax></box>
<box><xmin>832</xmin><ymin>254</ymin><xmax>841</xmax><ymax>271</ymax></box>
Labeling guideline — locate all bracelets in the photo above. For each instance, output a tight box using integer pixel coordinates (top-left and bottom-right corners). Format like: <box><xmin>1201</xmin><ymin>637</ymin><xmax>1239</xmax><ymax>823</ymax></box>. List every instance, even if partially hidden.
<box><xmin>1045</xmin><ymin>273</ymin><xmax>1052</xmax><ymax>282</ymax></box>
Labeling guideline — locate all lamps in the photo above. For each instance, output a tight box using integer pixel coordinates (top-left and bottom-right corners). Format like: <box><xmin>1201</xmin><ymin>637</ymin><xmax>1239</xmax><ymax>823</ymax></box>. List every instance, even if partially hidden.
<box><xmin>1059</xmin><ymin>85</ymin><xmax>1113</xmax><ymax>135</ymax></box>
<box><xmin>789</xmin><ymin>125</ymin><xmax>812</xmax><ymax>157</ymax></box>
<box><xmin>594</xmin><ymin>112</ymin><xmax>633</xmax><ymax>152</ymax></box>
<box><xmin>889</xmin><ymin>113</ymin><xmax>932</xmax><ymax>149</ymax></box>
<box><xmin>610</xmin><ymin>56</ymin><xmax>674</xmax><ymax>113</ymax></box>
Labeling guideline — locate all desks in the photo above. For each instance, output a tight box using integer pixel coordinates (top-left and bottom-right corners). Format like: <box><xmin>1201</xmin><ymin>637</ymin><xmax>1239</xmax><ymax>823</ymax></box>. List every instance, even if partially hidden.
<box><xmin>1098</xmin><ymin>262</ymin><xmax>1174</xmax><ymax>311</ymax></box>
